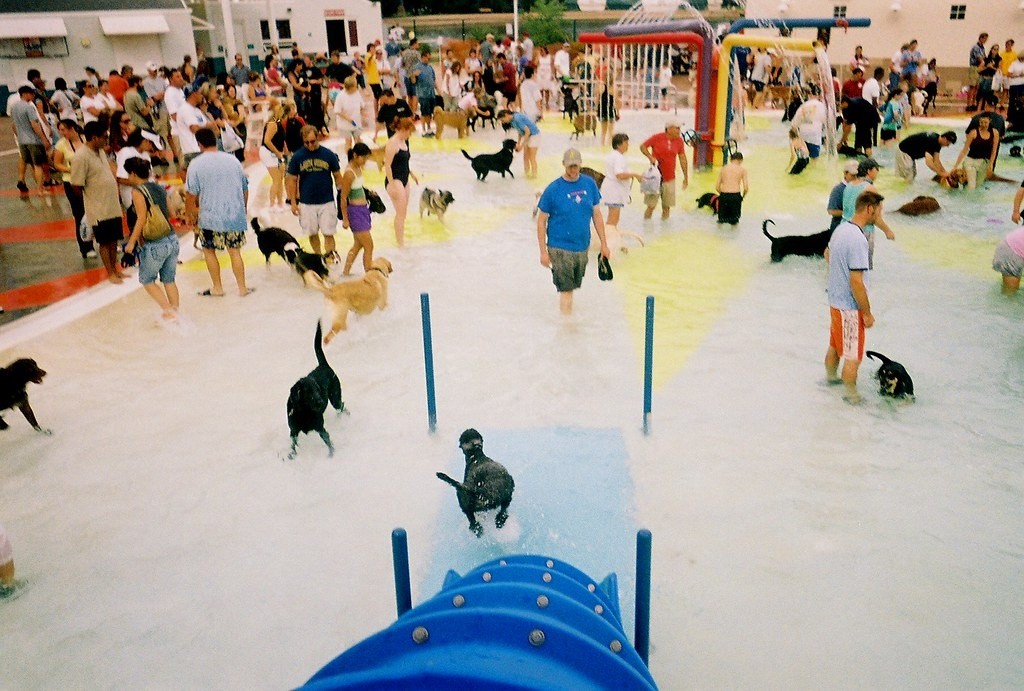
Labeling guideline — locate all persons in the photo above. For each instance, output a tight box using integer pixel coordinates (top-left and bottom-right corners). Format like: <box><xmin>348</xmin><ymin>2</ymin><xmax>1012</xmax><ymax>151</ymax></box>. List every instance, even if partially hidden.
<box><xmin>185</xmin><ymin>128</ymin><xmax>256</xmax><ymax>296</ymax></box>
<box><xmin>0</xmin><ymin>23</ymin><xmax>1024</xmax><ymax>318</ymax></box>
<box><xmin>821</xmin><ymin>191</ymin><xmax>886</xmax><ymax>407</ymax></box>
<box><xmin>537</xmin><ymin>147</ymin><xmax>610</xmax><ymax>318</ymax></box>
<box><xmin>122</xmin><ymin>156</ymin><xmax>180</xmax><ymax>318</ymax></box>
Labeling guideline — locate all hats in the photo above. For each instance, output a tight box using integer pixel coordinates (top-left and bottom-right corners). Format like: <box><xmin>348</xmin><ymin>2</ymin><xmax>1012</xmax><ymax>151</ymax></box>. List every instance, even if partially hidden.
<box><xmin>183</xmin><ymin>83</ymin><xmax>199</xmax><ymax>98</ymax></box>
<box><xmin>562</xmin><ymin>148</ymin><xmax>582</xmax><ymax>168</ymax></box>
<box><xmin>858</xmin><ymin>158</ymin><xmax>884</xmax><ymax>170</ymax></box>
<box><xmin>18</xmin><ymin>85</ymin><xmax>35</xmax><ymax>93</ymax></box>
<box><xmin>141</xmin><ymin>129</ymin><xmax>164</xmax><ymax>151</ymax></box>
<box><xmin>146</xmin><ymin>62</ymin><xmax>157</xmax><ymax>71</ymax></box>
<box><xmin>844</xmin><ymin>160</ymin><xmax>859</xmax><ymax>175</ymax></box>
<box><xmin>665</xmin><ymin>118</ymin><xmax>681</xmax><ymax>129</ymax></box>
<box><xmin>22</xmin><ymin>81</ymin><xmax>35</xmax><ymax>90</ymax></box>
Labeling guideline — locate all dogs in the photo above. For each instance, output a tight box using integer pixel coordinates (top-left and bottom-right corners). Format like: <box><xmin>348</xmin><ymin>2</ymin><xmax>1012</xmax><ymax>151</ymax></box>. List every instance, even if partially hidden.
<box><xmin>559</xmin><ymin>87</ymin><xmax>579</xmax><ymax>120</ymax></box>
<box><xmin>533</xmin><ymin>167</ymin><xmax>719</xmax><ymax>256</ymax></box>
<box><xmin>570</xmin><ymin>84</ymin><xmax>616</xmax><ymax>140</ymax></box>
<box><xmin>361</xmin><ymin>144</ymin><xmax>454</xmax><ymax>225</ymax></box>
<box><xmin>436</xmin><ymin>429</ymin><xmax>515</xmax><ymax>538</ymax></box>
<box><xmin>762</xmin><ymin>220</ymin><xmax>832</xmax><ymax>262</ymax></box>
<box><xmin>1009</xmin><ymin>146</ymin><xmax>1021</xmax><ymax>157</ymax></box>
<box><xmin>461</xmin><ymin>139</ymin><xmax>517</xmax><ymax>182</ymax></box>
<box><xmin>142</xmin><ymin>113</ymin><xmax>199</xmax><ymax>225</ymax></box>
<box><xmin>743</xmin><ymin>83</ymin><xmax>868</xmax><ymax>160</ymax></box>
<box><xmin>866</xmin><ymin>351</ymin><xmax>915</xmax><ymax>404</ymax></box>
<box><xmin>0</xmin><ymin>359</ymin><xmax>51</xmax><ymax>435</ymax></box>
<box><xmin>251</xmin><ymin>217</ymin><xmax>393</xmax><ymax>346</ymax></box>
<box><xmin>433</xmin><ymin>91</ymin><xmax>520</xmax><ymax>140</ymax></box>
<box><xmin>886</xmin><ymin>168</ymin><xmax>968</xmax><ymax>218</ymax></box>
<box><xmin>906</xmin><ymin>82</ymin><xmax>937</xmax><ymax>116</ymax></box>
<box><xmin>287</xmin><ymin>318</ymin><xmax>350</xmax><ymax>460</ymax></box>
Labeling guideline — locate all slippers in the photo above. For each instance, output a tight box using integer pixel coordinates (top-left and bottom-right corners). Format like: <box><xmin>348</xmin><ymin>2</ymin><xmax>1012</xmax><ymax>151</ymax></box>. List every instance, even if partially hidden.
<box><xmin>248</xmin><ymin>287</ymin><xmax>255</xmax><ymax>292</ymax></box>
<box><xmin>203</xmin><ymin>288</ymin><xmax>211</xmax><ymax>297</ymax></box>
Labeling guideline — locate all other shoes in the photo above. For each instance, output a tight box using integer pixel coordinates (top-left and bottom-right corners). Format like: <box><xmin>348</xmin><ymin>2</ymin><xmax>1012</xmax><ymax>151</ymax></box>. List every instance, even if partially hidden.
<box><xmin>17</xmin><ymin>180</ymin><xmax>29</xmax><ymax>193</ymax></box>
<box><xmin>421</xmin><ymin>132</ymin><xmax>430</xmax><ymax>138</ymax></box>
<box><xmin>598</xmin><ymin>253</ymin><xmax>613</xmax><ymax>281</ymax></box>
<box><xmin>87</xmin><ymin>250</ymin><xmax>97</xmax><ymax>259</ymax></box>
<box><xmin>428</xmin><ymin>131</ymin><xmax>435</xmax><ymax>137</ymax></box>
<box><xmin>154</xmin><ymin>317</ymin><xmax>177</xmax><ymax>326</ymax></box>
<box><xmin>43</xmin><ymin>178</ymin><xmax>59</xmax><ymax>187</ymax></box>
<box><xmin>160</xmin><ymin>157</ymin><xmax>169</xmax><ymax>166</ymax></box>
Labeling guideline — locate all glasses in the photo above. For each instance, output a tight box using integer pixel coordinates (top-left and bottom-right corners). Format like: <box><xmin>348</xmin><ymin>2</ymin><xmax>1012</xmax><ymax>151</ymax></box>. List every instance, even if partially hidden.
<box><xmin>88</xmin><ymin>84</ymin><xmax>94</xmax><ymax>88</ymax></box>
<box><xmin>121</xmin><ymin>119</ymin><xmax>130</xmax><ymax>124</ymax></box>
<box><xmin>303</xmin><ymin>139</ymin><xmax>316</xmax><ymax>145</ymax></box>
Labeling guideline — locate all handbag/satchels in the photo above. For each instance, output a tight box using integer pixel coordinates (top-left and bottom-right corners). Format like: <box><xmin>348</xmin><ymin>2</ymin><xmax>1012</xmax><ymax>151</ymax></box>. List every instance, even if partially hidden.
<box><xmin>79</xmin><ymin>214</ymin><xmax>95</xmax><ymax>242</ymax></box>
<box><xmin>136</xmin><ymin>184</ymin><xmax>171</xmax><ymax>240</ymax></box>
<box><xmin>336</xmin><ymin>187</ymin><xmax>386</xmax><ymax>220</ymax></box>
<box><xmin>639</xmin><ymin>159</ymin><xmax>662</xmax><ymax>195</ymax></box>
<box><xmin>220</xmin><ymin>121</ymin><xmax>244</xmax><ymax>153</ymax></box>
<box><xmin>991</xmin><ymin>70</ymin><xmax>1004</xmax><ymax>92</ymax></box>
